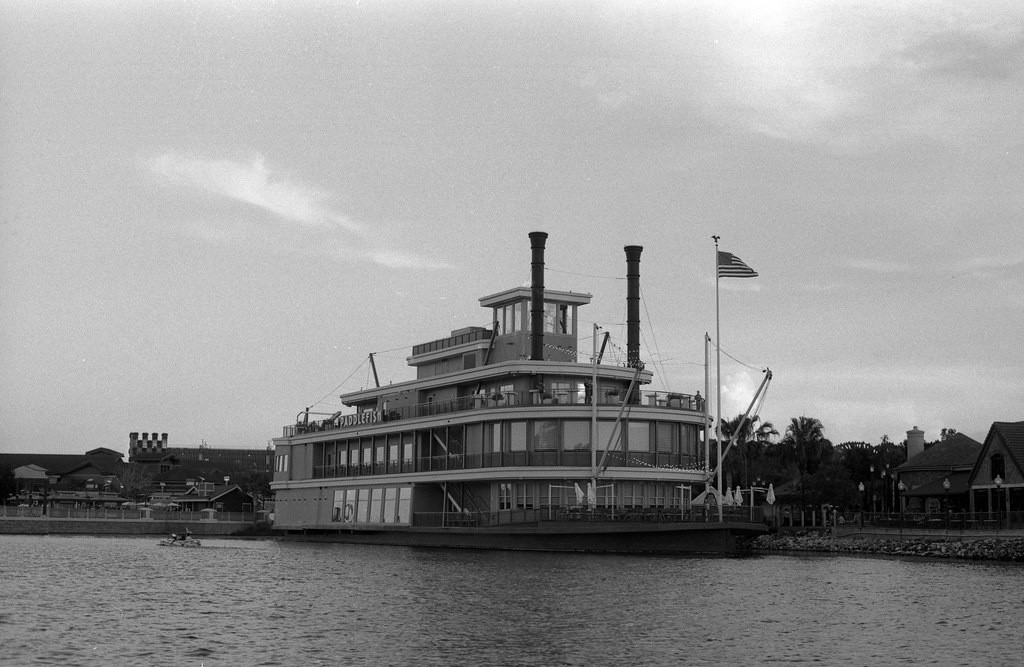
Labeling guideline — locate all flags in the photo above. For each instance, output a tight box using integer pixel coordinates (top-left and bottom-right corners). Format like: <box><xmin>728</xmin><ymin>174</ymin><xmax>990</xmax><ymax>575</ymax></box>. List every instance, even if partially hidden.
<box><xmin>716</xmin><ymin>252</ymin><xmax>758</xmax><ymax>278</ymax></box>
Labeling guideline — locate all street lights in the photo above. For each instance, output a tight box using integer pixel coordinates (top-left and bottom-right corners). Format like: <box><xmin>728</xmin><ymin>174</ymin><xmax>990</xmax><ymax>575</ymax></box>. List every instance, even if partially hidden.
<box><xmin>897</xmin><ymin>479</ymin><xmax>905</xmax><ymax>534</ymax></box>
<box><xmin>994</xmin><ymin>473</ymin><xmax>1003</xmax><ymax>528</ymax></box>
<box><xmin>858</xmin><ymin>481</ymin><xmax>865</xmax><ymax>527</ymax></box>
<box><xmin>943</xmin><ymin>477</ymin><xmax>951</xmax><ymax>535</ymax></box>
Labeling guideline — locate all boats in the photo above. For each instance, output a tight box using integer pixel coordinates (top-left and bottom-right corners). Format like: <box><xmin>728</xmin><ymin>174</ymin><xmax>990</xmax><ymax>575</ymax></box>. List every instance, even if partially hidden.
<box><xmin>157</xmin><ymin>526</ymin><xmax>202</xmax><ymax>548</ymax></box>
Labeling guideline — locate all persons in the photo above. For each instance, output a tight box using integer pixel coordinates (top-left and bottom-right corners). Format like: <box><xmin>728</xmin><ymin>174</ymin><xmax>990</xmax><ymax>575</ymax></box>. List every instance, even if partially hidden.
<box><xmin>536</xmin><ymin>380</ymin><xmax>544</xmax><ymax>402</ymax></box>
<box><xmin>695</xmin><ymin>391</ymin><xmax>701</xmax><ymax>411</ymax></box>
<box><xmin>584</xmin><ymin>377</ymin><xmax>593</xmax><ymax>404</ymax></box>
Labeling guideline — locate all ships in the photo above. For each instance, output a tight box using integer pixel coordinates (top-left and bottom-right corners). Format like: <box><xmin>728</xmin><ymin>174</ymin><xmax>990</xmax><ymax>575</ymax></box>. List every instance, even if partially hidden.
<box><xmin>264</xmin><ymin>230</ymin><xmax>778</xmax><ymax>557</ymax></box>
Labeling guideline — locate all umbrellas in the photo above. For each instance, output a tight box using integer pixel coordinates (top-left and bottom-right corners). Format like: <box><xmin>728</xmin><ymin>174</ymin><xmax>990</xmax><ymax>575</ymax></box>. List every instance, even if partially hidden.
<box><xmin>690</xmin><ymin>483</ymin><xmax>776</xmax><ymax>507</ymax></box>
<box><xmin>574</xmin><ymin>483</ymin><xmax>595</xmax><ymax>506</ymax></box>
